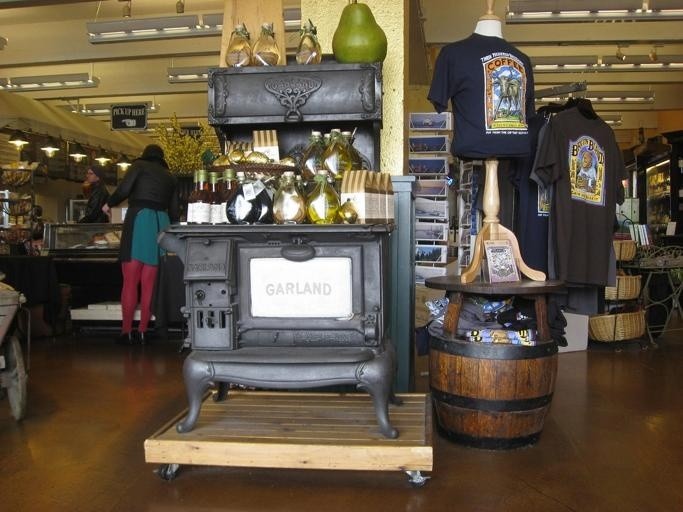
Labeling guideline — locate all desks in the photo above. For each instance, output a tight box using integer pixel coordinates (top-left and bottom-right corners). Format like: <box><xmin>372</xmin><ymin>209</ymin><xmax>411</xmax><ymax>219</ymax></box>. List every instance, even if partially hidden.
<box><xmin>0</xmin><ymin>254</ymin><xmax>57</xmax><ymax>340</ymax></box>
<box><xmin>424</xmin><ymin>275</ymin><xmax>569</xmax><ymax>451</ymax></box>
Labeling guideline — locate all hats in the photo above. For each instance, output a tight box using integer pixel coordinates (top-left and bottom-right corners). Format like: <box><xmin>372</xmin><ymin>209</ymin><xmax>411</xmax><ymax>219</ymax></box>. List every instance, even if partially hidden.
<box><xmin>90</xmin><ymin>165</ymin><xmax>104</xmax><ymax>180</ymax></box>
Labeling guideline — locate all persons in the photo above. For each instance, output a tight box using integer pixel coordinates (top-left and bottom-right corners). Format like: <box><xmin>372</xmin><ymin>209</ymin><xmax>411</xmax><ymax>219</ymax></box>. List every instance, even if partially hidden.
<box><xmin>78</xmin><ymin>165</ymin><xmax>111</xmax><ymax>223</ymax></box>
<box><xmin>101</xmin><ymin>144</ymin><xmax>180</xmax><ymax>348</ymax></box>
<box><xmin>431</xmin><ymin>13</ymin><xmax>538</xmax><ymax>161</ymax></box>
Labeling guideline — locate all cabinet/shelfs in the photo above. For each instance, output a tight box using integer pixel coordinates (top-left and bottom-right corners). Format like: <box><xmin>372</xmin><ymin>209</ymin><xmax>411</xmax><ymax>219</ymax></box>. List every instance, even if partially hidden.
<box><xmin>636</xmin><ymin>133</ymin><xmax>682</xmax><ymax>254</ymax></box>
<box><xmin>43</xmin><ymin>223</ymin><xmax>185</xmax><ymax>341</ymax></box>
<box><xmin>152</xmin><ymin>53</ymin><xmax>404</xmax><ymax>441</ymax></box>
<box><xmin>620</xmin><ymin>245</ymin><xmax>682</xmax><ymax>350</ymax></box>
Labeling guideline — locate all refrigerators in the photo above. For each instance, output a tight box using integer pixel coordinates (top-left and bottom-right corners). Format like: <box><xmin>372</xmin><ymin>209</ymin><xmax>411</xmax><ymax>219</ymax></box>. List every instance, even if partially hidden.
<box><xmin>633</xmin><ymin>129</ymin><xmax>682</xmax><ymax>337</ymax></box>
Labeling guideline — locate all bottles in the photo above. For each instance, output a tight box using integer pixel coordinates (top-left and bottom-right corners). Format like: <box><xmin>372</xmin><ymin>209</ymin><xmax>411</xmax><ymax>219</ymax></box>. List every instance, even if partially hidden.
<box><xmin>225</xmin><ymin>23</ymin><xmax>250</xmax><ymax>66</ymax></box>
<box><xmin>252</xmin><ymin>23</ymin><xmax>281</xmax><ymax>66</ymax></box>
<box><xmin>295</xmin><ymin>22</ymin><xmax>323</xmax><ymax>64</ymax></box>
<box><xmin>647</xmin><ymin>173</ymin><xmax>670</xmax><ymax>238</ymax></box>
<box><xmin>187</xmin><ymin>125</ymin><xmax>365</xmax><ymax>224</ymax></box>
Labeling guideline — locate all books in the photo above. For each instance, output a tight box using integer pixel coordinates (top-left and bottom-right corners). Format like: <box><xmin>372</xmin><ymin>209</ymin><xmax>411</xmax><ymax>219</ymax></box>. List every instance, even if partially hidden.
<box><xmin>628</xmin><ymin>223</ymin><xmax>654</xmax><ymax>250</ymax></box>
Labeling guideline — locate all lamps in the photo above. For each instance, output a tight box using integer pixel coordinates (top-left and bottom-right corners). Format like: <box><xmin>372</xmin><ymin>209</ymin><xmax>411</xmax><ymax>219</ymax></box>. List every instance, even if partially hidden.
<box><xmin>502</xmin><ymin>0</ymin><xmax>683</xmax><ymax>128</ymax></box>
<box><xmin>163</xmin><ymin>63</ymin><xmax>221</xmax><ymax>83</ymax></box>
<box><xmin>0</xmin><ymin>69</ymin><xmax>100</xmax><ymax>95</ymax></box>
<box><xmin>59</xmin><ymin>99</ymin><xmax>163</xmax><ymax>121</ymax></box>
<box><xmin>4</xmin><ymin>128</ymin><xmax>134</xmax><ymax>171</ymax></box>
<box><xmin>81</xmin><ymin>1</ymin><xmax>302</xmax><ymax>45</ymax></box>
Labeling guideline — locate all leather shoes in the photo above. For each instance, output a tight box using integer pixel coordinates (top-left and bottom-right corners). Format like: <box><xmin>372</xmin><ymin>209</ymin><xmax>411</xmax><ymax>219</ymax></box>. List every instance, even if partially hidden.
<box><xmin>114</xmin><ymin>331</ymin><xmax>132</xmax><ymax>345</ymax></box>
<box><xmin>132</xmin><ymin>332</ymin><xmax>147</xmax><ymax>345</ymax></box>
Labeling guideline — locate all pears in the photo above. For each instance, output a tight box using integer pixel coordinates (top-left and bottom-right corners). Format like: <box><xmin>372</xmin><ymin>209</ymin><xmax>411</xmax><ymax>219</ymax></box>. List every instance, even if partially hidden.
<box><xmin>332</xmin><ymin>0</ymin><xmax>387</xmax><ymax>64</ymax></box>
